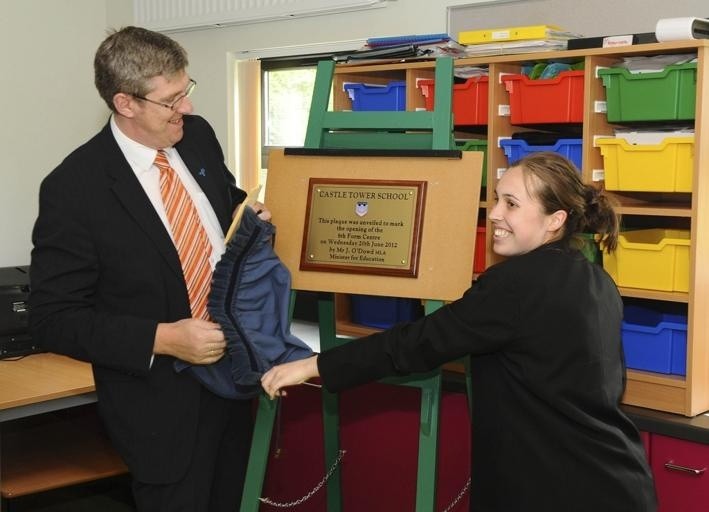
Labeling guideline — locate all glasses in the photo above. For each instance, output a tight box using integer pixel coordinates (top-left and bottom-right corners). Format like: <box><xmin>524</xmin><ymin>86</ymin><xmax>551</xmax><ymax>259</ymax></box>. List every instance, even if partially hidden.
<box><xmin>128</xmin><ymin>78</ymin><xmax>198</xmax><ymax>111</ymax></box>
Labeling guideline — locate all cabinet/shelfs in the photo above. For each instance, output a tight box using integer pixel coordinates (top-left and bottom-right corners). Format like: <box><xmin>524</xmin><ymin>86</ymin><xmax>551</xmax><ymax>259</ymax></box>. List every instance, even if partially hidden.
<box><xmin>329</xmin><ymin>39</ymin><xmax>709</xmax><ymax>416</ymax></box>
<box><xmin>263</xmin><ymin>375</ymin><xmax>709</xmax><ymax>511</ymax></box>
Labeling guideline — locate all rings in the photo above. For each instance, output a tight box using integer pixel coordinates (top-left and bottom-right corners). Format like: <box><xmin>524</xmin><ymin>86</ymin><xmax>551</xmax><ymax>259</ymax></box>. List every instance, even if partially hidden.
<box><xmin>209</xmin><ymin>350</ymin><xmax>214</xmax><ymax>357</ymax></box>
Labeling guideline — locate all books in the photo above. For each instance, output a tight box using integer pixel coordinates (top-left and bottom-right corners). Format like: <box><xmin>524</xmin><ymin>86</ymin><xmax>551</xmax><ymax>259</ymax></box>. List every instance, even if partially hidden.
<box><xmin>332</xmin><ymin>25</ymin><xmax>573</xmax><ymax>70</ymax></box>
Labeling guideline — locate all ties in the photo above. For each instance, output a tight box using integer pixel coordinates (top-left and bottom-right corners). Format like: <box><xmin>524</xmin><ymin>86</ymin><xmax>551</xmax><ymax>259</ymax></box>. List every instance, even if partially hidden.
<box><xmin>153</xmin><ymin>149</ymin><xmax>215</xmax><ymax>322</ymax></box>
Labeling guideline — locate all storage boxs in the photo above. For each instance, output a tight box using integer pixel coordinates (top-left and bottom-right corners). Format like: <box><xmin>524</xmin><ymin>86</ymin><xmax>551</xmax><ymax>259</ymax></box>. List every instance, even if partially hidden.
<box><xmin>499</xmin><ymin>138</ymin><xmax>582</xmax><ymax>175</ymax></box>
<box><xmin>500</xmin><ymin>69</ymin><xmax>585</xmax><ymax>125</ymax></box>
<box><xmin>417</xmin><ymin>74</ymin><xmax>490</xmax><ymax>125</ymax></box>
<box><xmin>344</xmin><ymin>81</ymin><xmax>404</xmax><ymax>111</ymax></box>
<box><xmin>455</xmin><ymin>139</ymin><xmax>487</xmax><ymax>186</ymax></box>
<box><xmin>620</xmin><ymin>304</ymin><xmax>687</xmax><ymax>374</ymax></box>
<box><xmin>596</xmin><ymin>137</ymin><xmax>695</xmax><ymax>194</ymax></box>
<box><xmin>471</xmin><ymin>227</ymin><xmax>486</xmax><ymax>273</ymax></box>
<box><xmin>597</xmin><ymin>63</ymin><xmax>698</xmax><ymax>124</ymax></box>
<box><xmin>594</xmin><ymin>226</ymin><xmax>690</xmax><ymax>295</ymax></box>
<box><xmin>347</xmin><ymin>294</ymin><xmax>421</xmax><ymax>331</ymax></box>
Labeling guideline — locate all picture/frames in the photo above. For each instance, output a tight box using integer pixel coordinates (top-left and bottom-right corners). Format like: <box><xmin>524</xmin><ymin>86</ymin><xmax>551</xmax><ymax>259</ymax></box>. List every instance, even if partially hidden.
<box><xmin>299</xmin><ymin>178</ymin><xmax>428</xmax><ymax>278</ymax></box>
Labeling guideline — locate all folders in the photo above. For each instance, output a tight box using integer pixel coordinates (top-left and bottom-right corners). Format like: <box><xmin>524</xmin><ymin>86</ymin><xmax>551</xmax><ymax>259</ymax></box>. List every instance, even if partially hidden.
<box><xmin>568</xmin><ymin>33</ymin><xmax>658</xmax><ymax>50</ymax></box>
<box><xmin>458</xmin><ymin>25</ymin><xmax>581</xmax><ymax>45</ymax></box>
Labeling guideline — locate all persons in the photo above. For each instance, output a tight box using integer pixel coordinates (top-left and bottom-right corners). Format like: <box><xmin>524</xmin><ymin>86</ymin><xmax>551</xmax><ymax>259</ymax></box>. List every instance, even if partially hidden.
<box><xmin>258</xmin><ymin>152</ymin><xmax>658</xmax><ymax>510</ymax></box>
<box><xmin>24</xmin><ymin>26</ymin><xmax>273</xmax><ymax>510</ymax></box>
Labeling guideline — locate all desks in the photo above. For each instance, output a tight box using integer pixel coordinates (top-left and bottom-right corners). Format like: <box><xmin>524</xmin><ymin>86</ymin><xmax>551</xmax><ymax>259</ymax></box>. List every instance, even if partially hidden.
<box><xmin>0</xmin><ymin>352</ymin><xmax>130</xmax><ymax>500</ymax></box>
